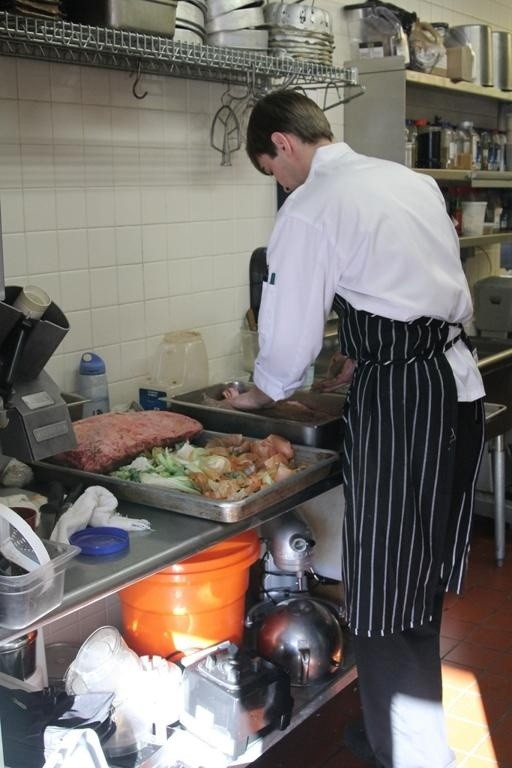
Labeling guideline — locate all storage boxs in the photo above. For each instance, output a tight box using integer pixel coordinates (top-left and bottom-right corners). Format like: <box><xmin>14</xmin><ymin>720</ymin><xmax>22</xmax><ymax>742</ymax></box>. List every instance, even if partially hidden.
<box><xmin>0</xmin><ymin>538</ymin><xmax>82</xmax><ymax>631</ymax></box>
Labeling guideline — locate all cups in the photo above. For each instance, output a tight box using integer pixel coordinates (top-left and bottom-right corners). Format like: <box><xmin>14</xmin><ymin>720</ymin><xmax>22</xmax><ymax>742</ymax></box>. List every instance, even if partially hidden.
<box><xmin>344</xmin><ymin>3</ymin><xmax>380</xmax><ymax>56</ymax></box>
<box><xmin>9</xmin><ymin>506</ymin><xmax>37</xmax><ymax>534</ymax></box>
<box><xmin>240</xmin><ymin>324</ymin><xmax>259</xmax><ymax>373</ymax></box>
<box><xmin>451</xmin><ymin>24</ymin><xmax>512</xmax><ymax>91</ymax></box>
<box><xmin>461</xmin><ymin>200</ymin><xmax>489</xmax><ymax>239</ymax></box>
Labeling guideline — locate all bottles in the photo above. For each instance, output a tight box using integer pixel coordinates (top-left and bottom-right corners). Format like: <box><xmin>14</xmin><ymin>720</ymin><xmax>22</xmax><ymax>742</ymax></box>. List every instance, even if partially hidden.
<box><xmin>405</xmin><ymin>116</ymin><xmax>509</xmax><ymax>172</ymax></box>
<box><xmin>77</xmin><ymin>353</ymin><xmax>110</xmax><ymax>415</ymax></box>
<box><xmin>139</xmin><ymin>329</ymin><xmax>210</xmax><ymax>413</ymax></box>
<box><xmin>1</xmin><ymin>632</ymin><xmax>39</xmax><ymax>681</ymax></box>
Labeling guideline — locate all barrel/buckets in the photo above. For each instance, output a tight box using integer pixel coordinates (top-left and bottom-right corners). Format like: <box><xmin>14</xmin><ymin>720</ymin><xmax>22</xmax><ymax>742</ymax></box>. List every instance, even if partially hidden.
<box><xmin>117</xmin><ymin>525</ymin><xmax>260</xmax><ymax>665</ymax></box>
<box><xmin>153</xmin><ymin>332</ymin><xmax>209</xmax><ymax>394</ymax></box>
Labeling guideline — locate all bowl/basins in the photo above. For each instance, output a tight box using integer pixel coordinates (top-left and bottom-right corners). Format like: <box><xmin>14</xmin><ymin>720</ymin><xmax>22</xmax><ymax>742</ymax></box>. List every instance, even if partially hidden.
<box><xmin>484</xmin><ymin>222</ymin><xmax>497</xmax><ymax>234</ymax></box>
<box><xmin>24</xmin><ymin>689</ymin><xmax>117</xmax><ymax>749</ymax></box>
<box><xmin>266</xmin><ymin>1</ymin><xmax>337</xmax><ymax>66</ymax></box>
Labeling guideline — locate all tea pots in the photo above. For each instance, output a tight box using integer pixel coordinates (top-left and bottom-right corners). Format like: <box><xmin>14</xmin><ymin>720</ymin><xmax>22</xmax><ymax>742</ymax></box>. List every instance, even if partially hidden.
<box><xmin>259</xmin><ymin>597</ymin><xmax>343</xmax><ymax>689</ymax></box>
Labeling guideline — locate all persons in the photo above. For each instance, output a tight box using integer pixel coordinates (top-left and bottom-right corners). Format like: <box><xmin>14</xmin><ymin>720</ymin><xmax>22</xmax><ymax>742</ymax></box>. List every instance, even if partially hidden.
<box><xmin>227</xmin><ymin>86</ymin><xmax>489</xmax><ymax>767</ymax></box>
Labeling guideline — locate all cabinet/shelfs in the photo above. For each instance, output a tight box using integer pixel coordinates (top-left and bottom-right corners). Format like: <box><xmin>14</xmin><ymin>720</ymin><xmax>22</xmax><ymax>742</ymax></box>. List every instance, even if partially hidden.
<box><xmin>343</xmin><ymin>56</ymin><xmax>512</xmax><ymax>367</ymax></box>
